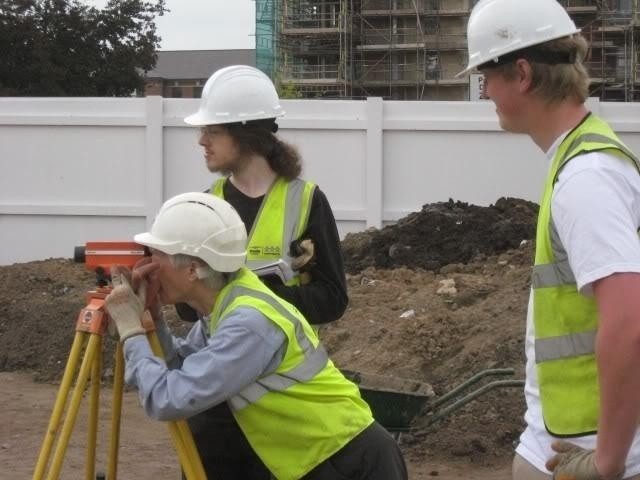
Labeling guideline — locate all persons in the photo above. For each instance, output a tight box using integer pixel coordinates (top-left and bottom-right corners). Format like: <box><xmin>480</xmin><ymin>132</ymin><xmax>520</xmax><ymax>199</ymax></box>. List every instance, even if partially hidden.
<box><xmin>453</xmin><ymin>0</ymin><xmax>640</xmax><ymax>478</ymax></box>
<box><xmin>173</xmin><ymin>62</ymin><xmax>349</xmax><ymax>479</ymax></box>
<box><xmin>103</xmin><ymin>190</ymin><xmax>408</xmax><ymax>479</ymax></box>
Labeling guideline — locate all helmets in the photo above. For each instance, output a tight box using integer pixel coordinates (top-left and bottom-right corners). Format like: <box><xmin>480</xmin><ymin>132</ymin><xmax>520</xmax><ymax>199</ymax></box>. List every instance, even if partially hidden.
<box><xmin>132</xmin><ymin>192</ymin><xmax>249</xmax><ymax>274</ymax></box>
<box><xmin>181</xmin><ymin>66</ymin><xmax>283</xmax><ymax>127</ymax></box>
<box><xmin>454</xmin><ymin>0</ymin><xmax>582</xmax><ymax>81</ymax></box>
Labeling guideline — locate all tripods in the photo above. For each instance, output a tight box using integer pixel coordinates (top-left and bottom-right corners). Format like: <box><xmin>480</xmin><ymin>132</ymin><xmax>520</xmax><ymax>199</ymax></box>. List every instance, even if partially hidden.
<box><xmin>30</xmin><ymin>300</ymin><xmax>212</xmax><ymax>480</ymax></box>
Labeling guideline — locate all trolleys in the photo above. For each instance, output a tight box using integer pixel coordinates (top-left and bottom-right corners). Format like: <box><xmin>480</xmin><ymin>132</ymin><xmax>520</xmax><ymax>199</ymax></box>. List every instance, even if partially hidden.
<box><xmin>338</xmin><ymin>368</ymin><xmax>526</xmax><ymax>445</ymax></box>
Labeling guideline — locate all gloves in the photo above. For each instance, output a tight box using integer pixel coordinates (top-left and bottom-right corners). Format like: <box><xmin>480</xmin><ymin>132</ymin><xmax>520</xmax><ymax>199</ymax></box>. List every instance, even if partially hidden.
<box><xmin>547</xmin><ymin>443</ymin><xmax>628</xmax><ymax>474</ymax></box>
<box><xmin>103</xmin><ymin>270</ymin><xmax>148</xmax><ymax>343</ymax></box>
